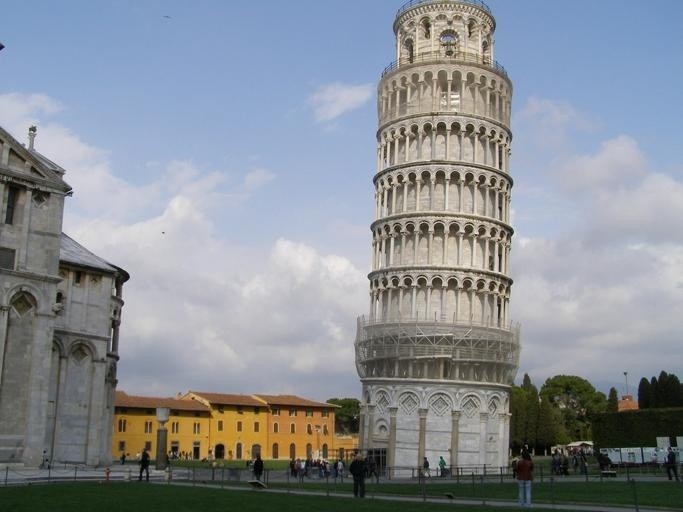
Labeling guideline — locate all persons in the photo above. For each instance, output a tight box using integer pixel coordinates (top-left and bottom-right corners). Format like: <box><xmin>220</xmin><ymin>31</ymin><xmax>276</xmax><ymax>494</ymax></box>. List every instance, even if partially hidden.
<box><xmin>664</xmin><ymin>447</ymin><xmax>679</xmax><ymax>481</ymax></box>
<box><xmin>512</xmin><ymin>454</ymin><xmax>520</xmax><ymax>479</ymax></box>
<box><xmin>422</xmin><ymin>457</ymin><xmax>430</xmax><ymax>477</ymax></box>
<box><xmin>169</xmin><ymin>450</ymin><xmax>193</xmax><ymax>461</ymax></box>
<box><xmin>349</xmin><ymin>455</ymin><xmax>378</xmax><ymax>498</ymax></box>
<box><xmin>290</xmin><ymin>455</ymin><xmax>345</xmax><ymax>484</ymax></box>
<box><xmin>551</xmin><ymin>447</ymin><xmax>589</xmax><ymax>475</ymax></box>
<box><xmin>138</xmin><ymin>449</ymin><xmax>150</xmax><ymax>481</ymax></box>
<box><xmin>438</xmin><ymin>456</ymin><xmax>447</xmax><ymax>477</ymax></box>
<box><xmin>254</xmin><ymin>455</ymin><xmax>263</xmax><ymax>480</ymax></box>
<box><xmin>517</xmin><ymin>450</ymin><xmax>534</xmax><ymax>505</ymax></box>
<box><xmin>40</xmin><ymin>450</ymin><xmax>50</xmax><ymax>469</ymax></box>
<box><xmin>120</xmin><ymin>454</ymin><xmax>126</xmax><ymax>464</ymax></box>
